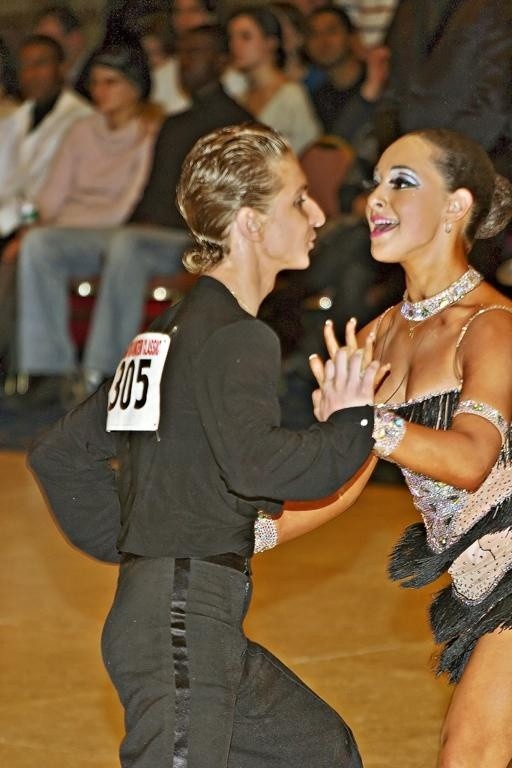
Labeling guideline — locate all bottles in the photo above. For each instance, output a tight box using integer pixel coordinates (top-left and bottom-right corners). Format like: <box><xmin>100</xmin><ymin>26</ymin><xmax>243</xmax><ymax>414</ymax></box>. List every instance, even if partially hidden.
<box><xmin>21</xmin><ymin>198</ymin><xmax>39</xmax><ymax>226</ymax></box>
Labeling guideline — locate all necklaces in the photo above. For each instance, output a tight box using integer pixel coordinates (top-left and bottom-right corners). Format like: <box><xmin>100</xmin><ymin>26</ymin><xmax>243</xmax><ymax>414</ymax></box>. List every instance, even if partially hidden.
<box><xmin>401</xmin><ymin>264</ymin><xmax>484</xmax><ymax>321</ymax></box>
<box><xmin>409</xmin><ymin>286</ymin><xmax>478</xmax><ymax>339</ymax></box>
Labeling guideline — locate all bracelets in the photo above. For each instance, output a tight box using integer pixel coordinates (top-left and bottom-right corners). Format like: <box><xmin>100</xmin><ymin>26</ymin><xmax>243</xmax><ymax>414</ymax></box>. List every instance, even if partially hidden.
<box><xmin>370</xmin><ymin>408</ymin><xmax>406</xmax><ymax>459</ymax></box>
<box><xmin>253</xmin><ymin>510</ymin><xmax>278</xmax><ymax>554</ymax></box>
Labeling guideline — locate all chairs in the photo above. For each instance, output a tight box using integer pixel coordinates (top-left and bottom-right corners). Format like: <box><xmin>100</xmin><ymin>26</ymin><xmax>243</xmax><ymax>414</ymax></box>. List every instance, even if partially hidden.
<box><xmin>69</xmin><ymin>136</ymin><xmax>355</xmax><ymax>349</ymax></box>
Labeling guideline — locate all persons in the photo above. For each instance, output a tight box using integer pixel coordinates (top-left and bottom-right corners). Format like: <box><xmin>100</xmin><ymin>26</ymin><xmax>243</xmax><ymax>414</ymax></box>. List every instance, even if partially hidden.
<box><xmin>0</xmin><ymin>0</ymin><xmax>510</xmax><ymax>377</ymax></box>
<box><xmin>28</xmin><ymin>122</ymin><xmax>381</xmax><ymax>768</ymax></box>
<box><xmin>254</xmin><ymin>130</ymin><xmax>512</xmax><ymax>768</ymax></box>
